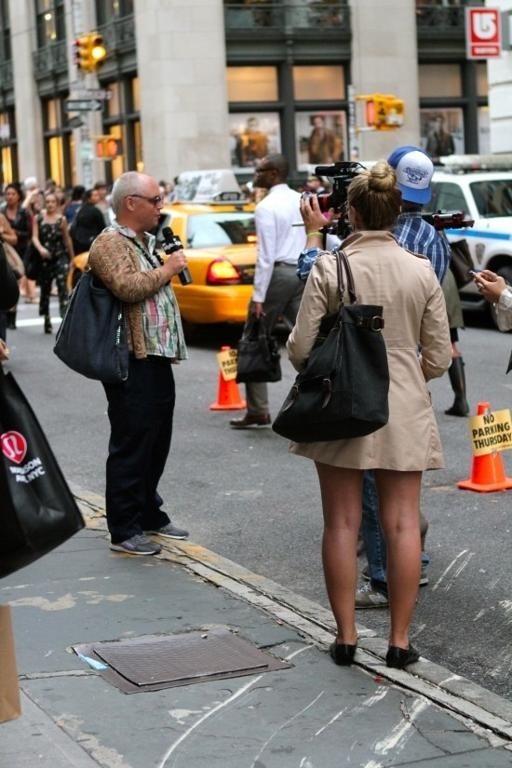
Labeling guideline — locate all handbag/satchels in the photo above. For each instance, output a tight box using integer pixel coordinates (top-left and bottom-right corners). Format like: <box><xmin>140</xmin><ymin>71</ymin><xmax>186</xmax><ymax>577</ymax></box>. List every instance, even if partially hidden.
<box><xmin>2</xmin><ymin>242</ymin><xmax>27</xmax><ymax>277</ymax></box>
<box><xmin>447</xmin><ymin>240</ymin><xmax>475</xmax><ymax>290</ymax></box>
<box><xmin>272</xmin><ymin>301</ymin><xmax>389</xmax><ymax>444</ymax></box>
<box><xmin>1</xmin><ymin>244</ymin><xmax>20</xmax><ymax>311</ymax></box>
<box><xmin>25</xmin><ymin>236</ymin><xmax>44</xmax><ymax>281</ymax></box>
<box><xmin>233</xmin><ymin>311</ymin><xmax>282</xmax><ymax>382</ymax></box>
<box><xmin>0</xmin><ymin>602</ymin><xmax>20</xmax><ymax>724</ymax></box>
<box><xmin>52</xmin><ymin>268</ymin><xmax>133</xmax><ymax>385</ymax></box>
<box><xmin>0</xmin><ymin>369</ymin><xmax>86</xmax><ymax>581</ymax></box>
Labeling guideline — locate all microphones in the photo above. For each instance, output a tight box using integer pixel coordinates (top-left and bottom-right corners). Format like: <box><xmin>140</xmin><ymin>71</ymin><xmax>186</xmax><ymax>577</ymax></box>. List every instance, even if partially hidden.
<box><xmin>160</xmin><ymin>227</ymin><xmax>192</xmax><ymax>286</ymax></box>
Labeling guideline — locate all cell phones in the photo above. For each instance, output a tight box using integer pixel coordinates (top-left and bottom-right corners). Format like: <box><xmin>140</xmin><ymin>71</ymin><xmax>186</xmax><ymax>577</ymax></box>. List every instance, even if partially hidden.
<box><xmin>469</xmin><ymin>270</ymin><xmax>482</xmax><ymax>279</ymax></box>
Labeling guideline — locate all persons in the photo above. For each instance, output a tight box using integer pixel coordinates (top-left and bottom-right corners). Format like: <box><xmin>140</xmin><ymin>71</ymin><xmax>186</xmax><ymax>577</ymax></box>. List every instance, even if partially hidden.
<box><xmin>425</xmin><ymin>113</ymin><xmax>455</xmax><ymax>157</ymax></box>
<box><xmin>442</xmin><ymin>267</ymin><xmax>470</xmax><ymax>416</ymax></box>
<box><xmin>1</xmin><ymin>176</ymin><xmax>186</xmax><ymax>368</ymax></box>
<box><xmin>294</xmin><ymin>145</ymin><xmax>451</xmax><ymax>608</ymax></box>
<box><xmin>285</xmin><ymin>161</ymin><xmax>451</xmax><ymax>667</ymax></box>
<box><xmin>308</xmin><ymin>116</ymin><xmax>342</xmax><ymax>164</ymax></box>
<box><xmin>474</xmin><ymin>270</ymin><xmax>512</xmax><ymax>373</ymax></box>
<box><xmin>230</xmin><ymin>164</ymin><xmax>341</xmax><ymax>429</ymax></box>
<box><xmin>235</xmin><ymin>117</ymin><xmax>269</xmax><ymax>167</ymax></box>
<box><xmin>87</xmin><ymin>170</ymin><xmax>189</xmax><ymax>558</ymax></box>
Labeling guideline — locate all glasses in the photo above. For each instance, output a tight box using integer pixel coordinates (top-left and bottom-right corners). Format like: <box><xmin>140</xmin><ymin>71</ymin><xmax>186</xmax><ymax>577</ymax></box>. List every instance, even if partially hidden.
<box><xmin>131</xmin><ymin>192</ymin><xmax>167</xmax><ymax>205</ymax></box>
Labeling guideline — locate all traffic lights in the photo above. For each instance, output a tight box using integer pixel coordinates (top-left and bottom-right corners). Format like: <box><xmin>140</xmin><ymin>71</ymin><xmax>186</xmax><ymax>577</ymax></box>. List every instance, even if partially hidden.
<box><xmin>75</xmin><ymin>34</ymin><xmax>93</xmax><ymax>72</ymax></box>
<box><xmin>104</xmin><ymin>137</ymin><xmax>122</xmax><ymax>160</ymax></box>
<box><xmin>95</xmin><ymin>135</ymin><xmax>106</xmax><ymax>158</ymax></box>
<box><xmin>363</xmin><ymin>96</ymin><xmax>404</xmax><ymax>131</ymax></box>
<box><xmin>88</xmin><ymin>33</ymin><xmax>108</xmax><ymax>72</ymax></box>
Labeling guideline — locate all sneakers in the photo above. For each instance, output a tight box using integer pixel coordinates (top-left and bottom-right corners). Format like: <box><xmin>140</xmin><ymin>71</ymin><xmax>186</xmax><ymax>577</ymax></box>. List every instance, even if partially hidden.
<box><xmin>150</xmin><ymin>518</ymin><xmax>192</xmax><ymax>542</ymax></box>
<box><xmin>109</xmin><ymin>531</ymin><xmax>164</xmax><ymax>556</ymax></box>
<box><xmin>355</xmin><ymin>536</ymin><xmax>430</xmax><ymax>609</ymax></box>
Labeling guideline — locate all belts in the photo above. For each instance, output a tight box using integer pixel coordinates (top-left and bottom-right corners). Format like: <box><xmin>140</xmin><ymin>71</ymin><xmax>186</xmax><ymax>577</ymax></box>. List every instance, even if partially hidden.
<box><xmin>274</xmin><ymin>260</ymin><xmax>297</xmax><ymax>268</ymax></box>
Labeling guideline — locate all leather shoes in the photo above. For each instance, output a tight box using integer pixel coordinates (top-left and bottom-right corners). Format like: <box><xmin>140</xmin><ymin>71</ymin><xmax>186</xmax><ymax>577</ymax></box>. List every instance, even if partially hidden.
<box><xmin>384</xmin><ymin>640</ymin><xmax>423</xmax><ymax>669</ymax></box>
<box><xmin>329</xmin><ymin>629</ymin><xmax>359</xmax><ymax>667</ymax></box>
<box><xmin>229</xmin><ymin>408</ymin><xmax>276</xmax><ymax>430</ymax></box>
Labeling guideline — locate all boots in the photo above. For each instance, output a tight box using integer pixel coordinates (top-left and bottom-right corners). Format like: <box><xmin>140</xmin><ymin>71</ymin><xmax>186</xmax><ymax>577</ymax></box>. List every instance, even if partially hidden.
<box><xmin>444</xmin><ymin>355</ymin><xmax>471</xmax><ymax>415</ymax></box>
<box><xmin>45</xmin><ymin>315</ymin><xmax>54</xmax><ymax>334</ymax></box>
<box><xmin>5</xmin><ymin>311</ymin><xmax>17</xmax><ymax>331</ymax></box>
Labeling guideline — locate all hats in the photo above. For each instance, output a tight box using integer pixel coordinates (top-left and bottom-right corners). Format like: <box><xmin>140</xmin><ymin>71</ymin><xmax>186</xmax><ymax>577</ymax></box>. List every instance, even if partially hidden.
<box><xmin>386</xmin><ymin>144</ymin><xmax>438</xmax><ymax>205</ymax></box>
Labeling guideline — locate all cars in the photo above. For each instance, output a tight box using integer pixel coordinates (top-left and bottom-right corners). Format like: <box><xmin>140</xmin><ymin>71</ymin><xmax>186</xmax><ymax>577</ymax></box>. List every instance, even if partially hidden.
<box><xmin>64</xmin><ymin>170</ymin><xmax>289</xmax><ymax>330</ymax></box>
<box><xmin>429</xmin><ymin>151</ymin><xmax>512</xmax><ymax>335</ymax></box>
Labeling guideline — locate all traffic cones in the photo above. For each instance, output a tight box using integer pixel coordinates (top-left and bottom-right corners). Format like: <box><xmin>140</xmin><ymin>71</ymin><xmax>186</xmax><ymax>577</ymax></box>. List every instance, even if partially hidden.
<box><xmin>207</xmin><ymin>345</ymin><xmax>248</xmax><ymax>410</ymax></box>
<box><xmin>454</xmin><ymin>399</ymin><xmax>511</xmax><ymax>491</ymax></box>
